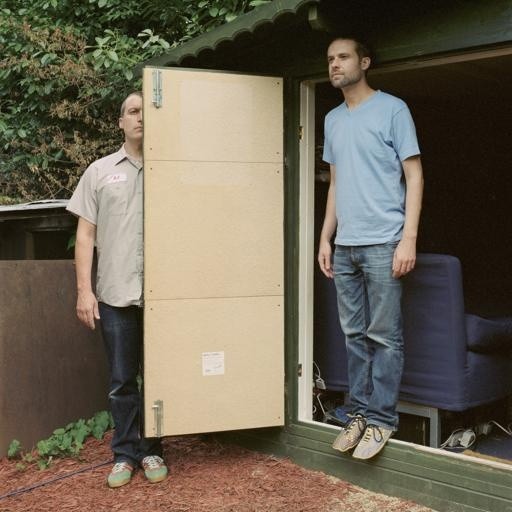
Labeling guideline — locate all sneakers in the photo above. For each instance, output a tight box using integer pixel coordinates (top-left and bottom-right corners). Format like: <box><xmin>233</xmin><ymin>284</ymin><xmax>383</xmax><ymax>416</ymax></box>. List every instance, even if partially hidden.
<box><xmin>140</xmin><ymin>455</ymin><xmax>168</xmax><ymax>484</ymax></box>
<box><xmin>352</xmin><ymin>424</ymin><xmax>393</xmax><ymax>459</ymax></box>
<box><xmin>107</xmin><ymin>462</ymin><xmax>133</xmax><ymax>488</ymax></box>
<box><xmin>332</xmin><ymin>413</ymin><xmax>367</xmax><ymax>453</ymax></box>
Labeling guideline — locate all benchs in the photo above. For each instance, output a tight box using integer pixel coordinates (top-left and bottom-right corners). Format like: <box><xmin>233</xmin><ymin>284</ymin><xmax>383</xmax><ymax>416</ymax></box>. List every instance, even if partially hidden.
<box><xmin>314</xmin><ymin>253</ymin><xmax>512</xmax><ymax>447</ymax></box>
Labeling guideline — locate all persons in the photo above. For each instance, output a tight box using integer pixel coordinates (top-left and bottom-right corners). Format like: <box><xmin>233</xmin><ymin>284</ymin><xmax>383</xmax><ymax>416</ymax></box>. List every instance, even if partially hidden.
<box><xmin>65</xmin><ymin>90</ymin><xmax>169</xmax><ymax>488</ymax></box>
<box><xmin>317</xmin><ymin>36</ymin><xmax>424</xmax><ymax>460</ymax></box>
